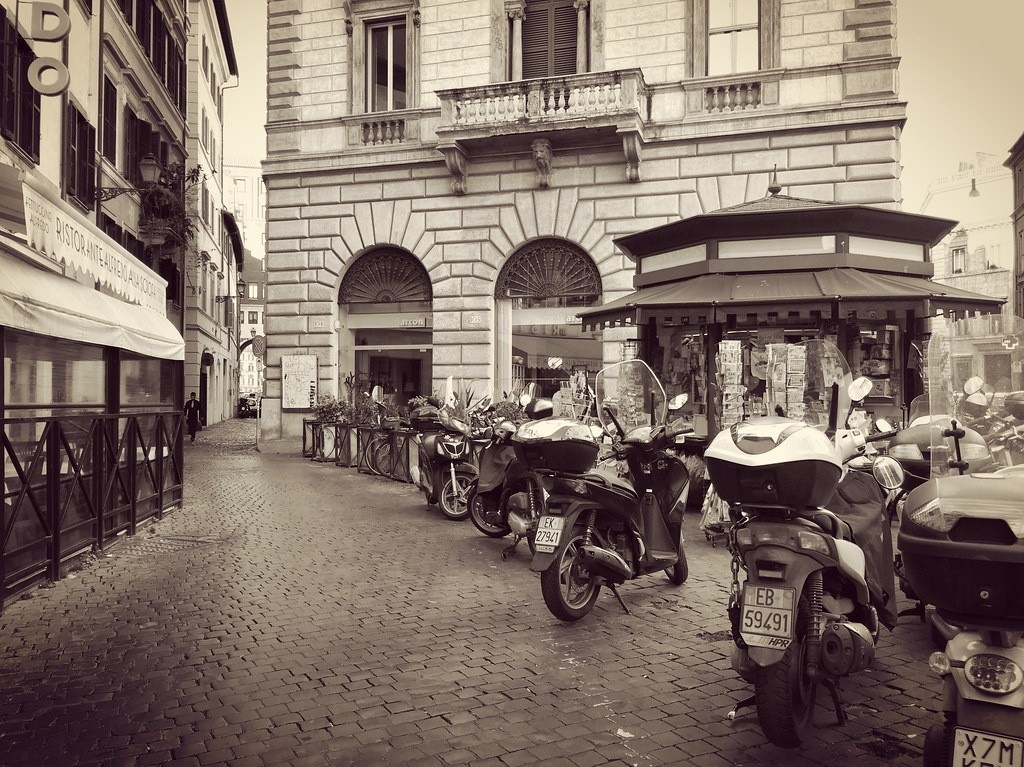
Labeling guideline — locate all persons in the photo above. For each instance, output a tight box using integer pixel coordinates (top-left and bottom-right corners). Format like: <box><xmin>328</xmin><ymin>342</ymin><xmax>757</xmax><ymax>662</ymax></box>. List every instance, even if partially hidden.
<box><xmin>184</xmin><ymin>392</ymin><xmax>204</xmax><ymax>443</ymax></box>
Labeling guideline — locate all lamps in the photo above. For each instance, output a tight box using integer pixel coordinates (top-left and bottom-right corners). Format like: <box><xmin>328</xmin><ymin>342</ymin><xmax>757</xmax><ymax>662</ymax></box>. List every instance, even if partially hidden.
<box><xmin>90</xmin><ymin>152</ymin><xmax>163</xmax><ymax>201</ymax></box>
<box><xmin>250</xmin><ymin>327</ymin><xmax>256</xmax><ymax>338</ymax></box>
<box><xmin>216</xmin><ymin>279</ymin><xmax>246</xmax><ymax>304</ymax></box>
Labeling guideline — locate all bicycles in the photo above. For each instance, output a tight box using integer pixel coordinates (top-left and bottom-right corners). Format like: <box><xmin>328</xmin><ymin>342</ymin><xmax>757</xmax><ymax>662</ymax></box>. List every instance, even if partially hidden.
<box><xmin>363</xmin><ymin>426</ymin><xmax>400</xmax><ymax>477</ymax></box>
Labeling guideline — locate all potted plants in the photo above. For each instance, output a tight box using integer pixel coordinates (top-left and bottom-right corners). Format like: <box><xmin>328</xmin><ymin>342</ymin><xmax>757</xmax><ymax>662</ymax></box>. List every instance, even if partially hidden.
<box><xmin>304</xmin><ymin>395</ymin><xmax>443</xmax><ymax>486</ymax></box>
<box><xmin>138</xmin><ymin>161</ymin><xmax>217</xmax><ymax>267</ymax></box>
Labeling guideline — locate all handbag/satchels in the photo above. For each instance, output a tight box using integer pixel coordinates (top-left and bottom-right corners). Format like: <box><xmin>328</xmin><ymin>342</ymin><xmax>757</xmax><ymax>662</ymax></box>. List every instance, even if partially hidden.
<box><xmin>197</xmin><ymin>421</ymin><xmax>202</xmax><ymax>431</ymax></box>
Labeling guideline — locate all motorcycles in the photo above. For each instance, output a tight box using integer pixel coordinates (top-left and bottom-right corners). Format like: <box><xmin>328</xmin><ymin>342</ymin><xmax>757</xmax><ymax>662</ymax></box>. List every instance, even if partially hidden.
<box><xmin>865</xmin><ymin>370</ymin><xmax>1024</xmax><ymax>481</ymax></box>
<box><xmin>891</xmin><ymin>460</ymin><xmax>1024</xmax><ymax>767</ymax></box>
<box><xmin>237</xmin><ymin>397</ymin><xmax>250</xmax><ymax>418</ymax></box>
<box><xmin>704</xmin><ymin>373</ymin><xmax>891</xmax><ymax>752</ymax></box>
<box><xmin>407</xmin><ymin>397</ymin><xmax>481</xmax><ymax>522</ymax></box>
<box><xmin>513</xmin><ymin>359</ymin><xmax>691</xmax><ymax>621</ymax></box>
<box><xmin>467</xmin><ymin>381</ymin><xmax>547</xmax><ymax>559</ymax></box>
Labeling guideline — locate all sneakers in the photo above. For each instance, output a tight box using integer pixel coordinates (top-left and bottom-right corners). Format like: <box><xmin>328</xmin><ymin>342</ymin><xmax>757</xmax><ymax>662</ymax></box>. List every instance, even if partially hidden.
<box><xmin>191</xmin><ymin>439</ymin><xmax>195</xmax><ymax>446</ymax></box>
